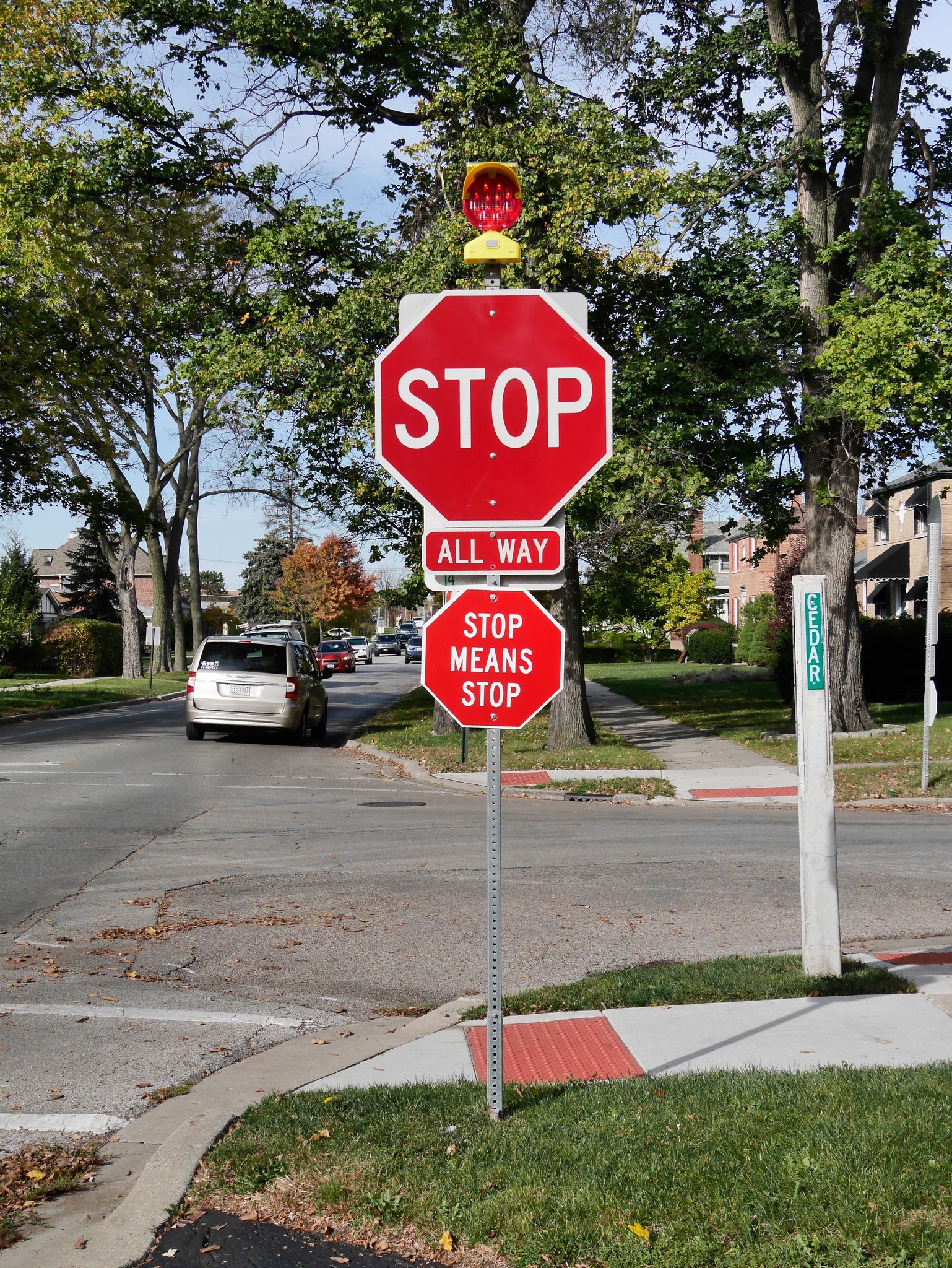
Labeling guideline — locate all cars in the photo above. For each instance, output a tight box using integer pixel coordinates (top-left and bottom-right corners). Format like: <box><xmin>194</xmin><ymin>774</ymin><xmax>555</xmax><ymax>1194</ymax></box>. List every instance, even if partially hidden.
<box><xmin>312</xmin><ymin>640</ymin><xmax>356</xmax><ymax>673</ymax></box>
<box><xmin>341</xmin><ymin>636</ymin><xmax>373</xmax><ymax>665</ymax></box>
<box><xmin>406</xmin><ymin>633</ymin><xmax>419</xmax><ymax>638</ymax></box>
<box><xmin>324</xmin><ymin>628</ymin><xmax>352</xmax><ymax>637</ymax></box>
<box><xmin>185</xmin><ymin>634</ymin><xmax>333</xmax><ymax>743</ymax></box>
<box><xmin>394</xmin><ymin>633</ymin><xmax>409</xmax><ymax>650</ymax></box>
<box><xmin>405</xmin><ymin>637</ymin><xmax>422</xmax><ymax>664</ymax></box>
<box><xmin>405</xmin><ymin>621</ymin><xmax>415</xmax><ymax>623</ymax></box>
<box><xmin>412</xmin><ymin>617</ymin><xmax>426</xmax><ymax>627</ymax></box>
<box><xmin>399</xmin><ymin>623</ymin><xmax>418</xmax><ymax>634</ymax></box>
<box><xmin>373</xmin><ymin>634</ymin><xmax>402</xmax><ymax>657</ymax></box>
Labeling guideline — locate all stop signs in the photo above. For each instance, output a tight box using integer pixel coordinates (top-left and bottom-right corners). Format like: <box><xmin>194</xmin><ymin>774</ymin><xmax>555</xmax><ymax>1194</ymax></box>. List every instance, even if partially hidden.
<box><xmin>420</xmin><ymin>585</ymin><xmax>565</xmax><ymax>729</ymax></box>
<box><xmin>375</xmin><ymin>288</ymin><xmax>613</xmax><ymax>528</ymax></box>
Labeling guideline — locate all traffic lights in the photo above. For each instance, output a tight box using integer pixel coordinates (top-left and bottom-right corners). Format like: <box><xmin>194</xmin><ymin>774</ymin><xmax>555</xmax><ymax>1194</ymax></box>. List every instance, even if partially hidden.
<box><xmin>457</xmin><ymin>163</ymin><xmax>523</xmax><ymax>234</ymax></box>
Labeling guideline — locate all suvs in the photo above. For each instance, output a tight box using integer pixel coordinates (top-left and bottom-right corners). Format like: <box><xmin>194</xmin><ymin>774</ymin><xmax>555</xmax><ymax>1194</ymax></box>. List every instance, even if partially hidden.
<box><xmin>234</xmin><ymin>620</ymin><xmax>303</xmax><ymax>646</ymax></box>
